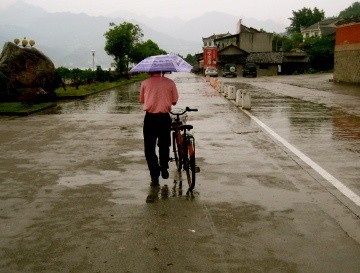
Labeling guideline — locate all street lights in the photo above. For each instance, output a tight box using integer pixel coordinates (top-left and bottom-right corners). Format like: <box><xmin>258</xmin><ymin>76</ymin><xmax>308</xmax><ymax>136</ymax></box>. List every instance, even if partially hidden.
<box><xmin>91</xmin><ymin>50</ymin><xmax>96</xmax><ymax>70</ymax></box>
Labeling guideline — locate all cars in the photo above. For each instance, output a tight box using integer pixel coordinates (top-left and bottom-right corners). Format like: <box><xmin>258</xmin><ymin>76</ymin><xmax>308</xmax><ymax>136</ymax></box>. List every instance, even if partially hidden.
<box><xmin>204</xmin><ymin>67</ymin><xmax>218</xmax><ymax>77</ymax></box>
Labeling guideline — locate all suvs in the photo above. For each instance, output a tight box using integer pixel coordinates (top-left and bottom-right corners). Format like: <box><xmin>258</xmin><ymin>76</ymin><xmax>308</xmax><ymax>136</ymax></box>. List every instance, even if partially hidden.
<box><xmin>243</xmin><ymin>62</ymin><xmax>257</xmax><ymax>77</ymax></box>
<box><xmin>222</xmin><ymin>64</ymin><xmax>237</xmax><ymax>77</ymax></box>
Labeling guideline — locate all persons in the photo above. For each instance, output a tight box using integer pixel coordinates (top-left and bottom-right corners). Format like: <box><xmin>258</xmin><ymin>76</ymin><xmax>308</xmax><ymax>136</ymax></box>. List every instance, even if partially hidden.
<box><xmin>140</xmin><ymin>72</ymin><xmax>179</xmax><ymax>188</ymax></box>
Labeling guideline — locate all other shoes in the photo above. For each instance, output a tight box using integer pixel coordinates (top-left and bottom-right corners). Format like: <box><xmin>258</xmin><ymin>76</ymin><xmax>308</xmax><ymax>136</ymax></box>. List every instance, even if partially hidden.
<box><xmin>159</xmin><ymin>163</ymin><xmax>170</xmax><ymax>179</ymax></box>
<box><xmin>150</xmin><ymin>171</ymin><xmax>160</xmax><ymax>186</ymax></box>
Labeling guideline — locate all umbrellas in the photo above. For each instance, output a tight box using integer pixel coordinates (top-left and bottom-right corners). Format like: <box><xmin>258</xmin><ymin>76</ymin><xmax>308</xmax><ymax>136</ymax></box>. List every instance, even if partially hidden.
<box><xmin>128</xmin><ymin>53</ymin><xmax>194</xmax><ymax>73</ymax></box>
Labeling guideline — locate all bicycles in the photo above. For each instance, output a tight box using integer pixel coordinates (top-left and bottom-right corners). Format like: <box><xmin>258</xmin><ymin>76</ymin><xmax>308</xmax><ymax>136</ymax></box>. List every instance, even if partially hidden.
<box><xmin>169</xmin><ymin>106</ymin><xmax>200</xmax><ymax>190</ymax></box>
